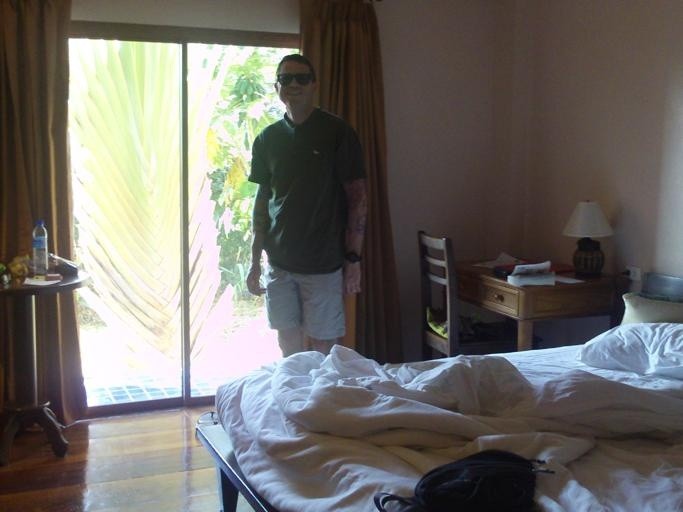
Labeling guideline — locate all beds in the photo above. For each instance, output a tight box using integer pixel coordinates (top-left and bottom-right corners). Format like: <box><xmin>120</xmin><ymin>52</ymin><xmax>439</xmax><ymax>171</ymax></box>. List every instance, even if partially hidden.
<box><xmin>196</xmin><ymin>270</ymin><xmax>682</xmax><ymax>512</ymax></box>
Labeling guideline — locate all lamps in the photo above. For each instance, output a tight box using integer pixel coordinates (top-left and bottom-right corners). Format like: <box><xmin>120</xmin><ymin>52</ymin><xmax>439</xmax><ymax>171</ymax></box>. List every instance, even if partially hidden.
<box><xmin>562</xmin><ymin>201</ymin><xmax>614</xmax><ymax>278</ymax></box>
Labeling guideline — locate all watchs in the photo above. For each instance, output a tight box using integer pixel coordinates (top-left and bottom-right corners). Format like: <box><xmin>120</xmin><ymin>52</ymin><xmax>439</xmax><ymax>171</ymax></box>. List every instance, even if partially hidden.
<box><xmin>345</xmin><ymin>251</ymin><xmax>362</xmax><ymax>263</ymax></box>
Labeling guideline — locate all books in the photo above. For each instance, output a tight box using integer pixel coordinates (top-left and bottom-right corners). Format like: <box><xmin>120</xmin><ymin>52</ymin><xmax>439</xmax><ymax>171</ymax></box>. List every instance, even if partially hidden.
<box><xmin>472</xmin><ymin>247</ymin><xmax>585</xmax><ymax>287</ymax></box>
<box><xmin>48</xmin><ymin>253</ymin><xmax>79</xmax><ymax>271</ymax></box>
<box><xmin>21</xmin><ymin>277</ymin><xmax>61</xmax><ymax>287</ymax></box>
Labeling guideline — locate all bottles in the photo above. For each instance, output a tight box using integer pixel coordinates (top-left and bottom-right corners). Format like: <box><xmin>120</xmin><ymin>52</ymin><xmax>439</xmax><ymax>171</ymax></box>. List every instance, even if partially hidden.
<box><xmin>31</xmin><ymin>218</ymin><xmax>49</xmax><ymax>275</ymax></box>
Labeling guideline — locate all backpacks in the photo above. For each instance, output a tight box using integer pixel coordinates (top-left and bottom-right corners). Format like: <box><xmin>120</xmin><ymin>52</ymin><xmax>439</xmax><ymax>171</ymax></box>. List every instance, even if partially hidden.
<box><xmin>372</xmin><ymin>449</ymin><xmax>555</xmax><ymax>512</ymax></box>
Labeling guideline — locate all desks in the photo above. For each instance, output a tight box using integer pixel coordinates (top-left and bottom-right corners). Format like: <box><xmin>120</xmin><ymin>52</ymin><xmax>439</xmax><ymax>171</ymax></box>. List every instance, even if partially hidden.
<box><xmin>1</xmin><ymin>270</ymin><xmax>90</xmax><ymax>458</ymax></box>
<box><xmin>442</xmin><ymin>258</ymin><xmax>631</xmax><ymax>351</ymax></box>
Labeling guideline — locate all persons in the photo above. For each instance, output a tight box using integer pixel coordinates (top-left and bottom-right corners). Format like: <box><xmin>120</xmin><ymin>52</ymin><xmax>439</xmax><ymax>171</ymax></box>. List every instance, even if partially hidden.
<box><xmin>246</xmin><ymin>53</ymin><xmax>370</xmax><ymax>359</ymax></box>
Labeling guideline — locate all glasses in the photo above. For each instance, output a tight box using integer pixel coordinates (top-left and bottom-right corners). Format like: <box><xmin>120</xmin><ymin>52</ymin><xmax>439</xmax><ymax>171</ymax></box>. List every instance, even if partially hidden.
<box><xmin>275</xmin><ymin>72</ymin><xmax>315</xmax><ymax>87</ymax></box>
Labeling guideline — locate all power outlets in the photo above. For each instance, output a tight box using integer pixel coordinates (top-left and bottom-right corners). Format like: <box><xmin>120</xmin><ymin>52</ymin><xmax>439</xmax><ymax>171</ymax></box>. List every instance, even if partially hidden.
<box><xmin>624</xmin><ymin>265</ymin><xmax>641</xmax><ymax>282</ymax></box>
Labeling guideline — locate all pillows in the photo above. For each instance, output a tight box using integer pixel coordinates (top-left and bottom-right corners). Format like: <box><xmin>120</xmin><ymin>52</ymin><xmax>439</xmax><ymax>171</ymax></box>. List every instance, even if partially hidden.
<box><xmin>619</xmin><ymin>290</ymin><xmax>682</xmax><ymax>325</ymax></box>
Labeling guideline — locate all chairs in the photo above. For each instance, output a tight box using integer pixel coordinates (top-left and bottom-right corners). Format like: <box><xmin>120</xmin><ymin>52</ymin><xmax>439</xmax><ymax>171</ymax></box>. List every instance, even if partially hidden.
<box><xmin>416</xmin><ymin>230</ymin><xmax>541</xmax><ymax>363</ymax></box>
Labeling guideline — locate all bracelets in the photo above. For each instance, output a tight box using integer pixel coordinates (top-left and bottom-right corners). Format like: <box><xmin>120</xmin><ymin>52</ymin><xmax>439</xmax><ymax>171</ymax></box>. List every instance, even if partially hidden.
<box><xmin>346</xmin><ymin>246</ymin><xmax>364</xmax><ymax>250</ymax></box>
